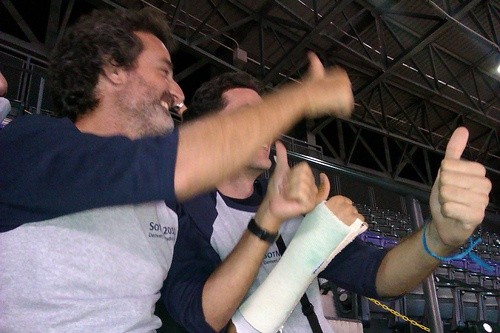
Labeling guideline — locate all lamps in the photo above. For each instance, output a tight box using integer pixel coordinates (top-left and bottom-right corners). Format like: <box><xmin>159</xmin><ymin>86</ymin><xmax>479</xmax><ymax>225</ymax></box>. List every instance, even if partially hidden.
<box><xmin>495</xmin><ymin>54</ymin><xmax>500</xmax><ymax>73</ymax></box>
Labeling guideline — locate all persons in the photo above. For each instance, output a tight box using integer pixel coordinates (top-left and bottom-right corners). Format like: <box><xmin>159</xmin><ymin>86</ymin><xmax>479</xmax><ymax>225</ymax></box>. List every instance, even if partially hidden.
<box><xmin>0</xmin><ymin>7</ymin><xmax>368</xmax><ymax>333</ymax></box>
<box><xmin>160</xmin><ymin>72</ymin><xmax>492</xmax><ymax>333</ymax></box>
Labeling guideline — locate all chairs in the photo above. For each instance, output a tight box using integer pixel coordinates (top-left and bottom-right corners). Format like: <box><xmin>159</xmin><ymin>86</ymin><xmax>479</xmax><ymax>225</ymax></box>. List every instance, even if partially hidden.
<box><xmin>319</xmin><ymin>201</ymin><xmax>499</xmax><ymax>333</ymax></box>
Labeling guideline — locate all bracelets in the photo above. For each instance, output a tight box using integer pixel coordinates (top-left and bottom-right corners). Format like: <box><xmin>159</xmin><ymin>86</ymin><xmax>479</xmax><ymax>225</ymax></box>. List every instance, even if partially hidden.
<box><xmin>247</xmin><ymin>216</ymin><xmax>279</xmax><ymax>245</ymax></box>
<box><xmin>421</xmin><ymin>216</ymin><xmax>491</xmax><ymax>274</ymax></box>
